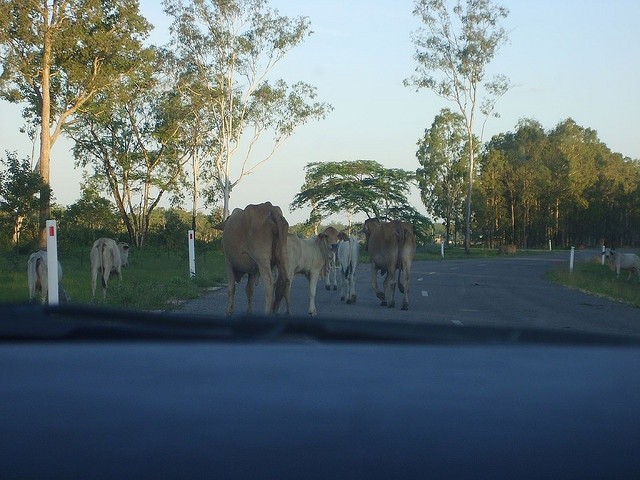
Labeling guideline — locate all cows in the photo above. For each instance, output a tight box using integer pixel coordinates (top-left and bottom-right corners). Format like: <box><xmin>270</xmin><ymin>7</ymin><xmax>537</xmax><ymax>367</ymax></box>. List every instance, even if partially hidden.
<box><xmin>362</xmin><ymin>217</ymin><xmax>416</xmax><ymax>311</ymax></box>
<box><xmin>90</xmin><ymin>237</ymin><xmax>129</xmax><ymax>301</ymax></box>
<box><xmin>275</xmin><ymin>226</ymin><xmax>349</xmax><ymax>317</ymax></box>
<box><xmin>323</xmin><ymin>232</ymin><xmax>359</xmax><ymax>303</ymax></box>
<box><xmin>26</xmin><ymin>250</ymin><xmax>63</xmax><ymax>306</ymax></box>
<box><xmin>601</xmin><ymin>247</ymin><xmax>640</xmax><ymax>284</ymax></box>
<box><xmin>495</xmin><ymin>242</ymin><xmax>519</xmax><ymax>255</ymax></box>
<box><xmin>211</xmin><ymin>201</ymin><xmax>294</xmax><ymax>316</ymax></box>
